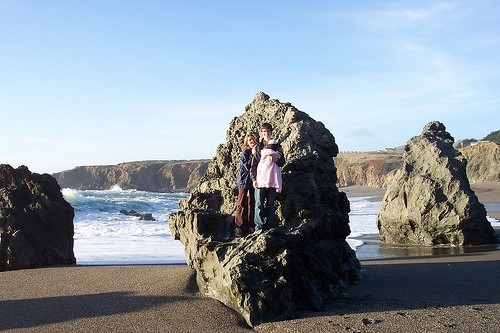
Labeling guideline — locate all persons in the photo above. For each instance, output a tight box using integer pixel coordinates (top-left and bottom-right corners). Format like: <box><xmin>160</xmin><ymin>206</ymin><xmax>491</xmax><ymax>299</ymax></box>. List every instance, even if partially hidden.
<box><xmin>258</xmin><ymin>139</ymin><xmax>282</xmax><ymax>186</ymax></box>
<box><xmin>250</xmin><ymin>122</ymin><xmax>285</xmax><ymax>236</ymax></box>
<box><xmin>233</xmin><ymin>134</ymin><xmax>262</xmax><ymax>238</ymax></box>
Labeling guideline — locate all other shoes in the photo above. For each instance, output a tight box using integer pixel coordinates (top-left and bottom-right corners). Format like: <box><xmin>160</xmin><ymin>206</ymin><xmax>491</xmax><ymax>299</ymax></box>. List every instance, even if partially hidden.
<box><xmin>255</xmin><ymin>226</ymin><xmax>273</xmax><ymax>231</ymax></box>
<box><xmin>247</xmin><ymin>228</ymin><xmax>255</xmax><ymax>234</ymax></box>
<box><xmin>234</xmin><ymin>228</ymin><xmax>245</xmax><ymax>236</ymax></box>
